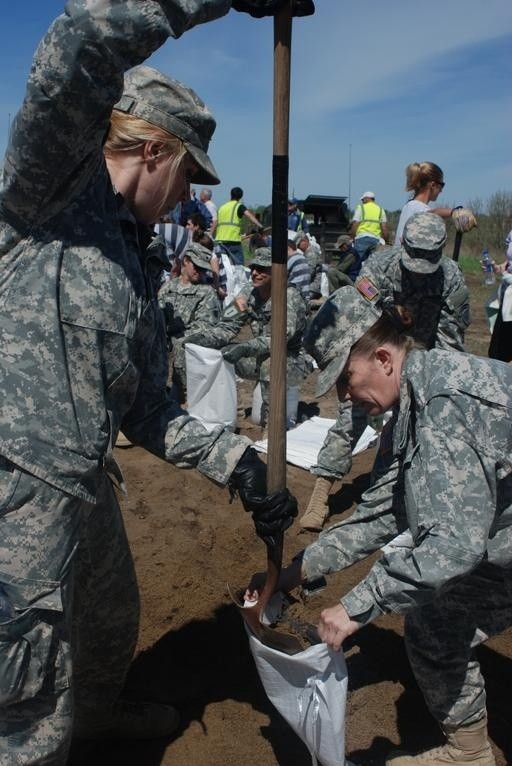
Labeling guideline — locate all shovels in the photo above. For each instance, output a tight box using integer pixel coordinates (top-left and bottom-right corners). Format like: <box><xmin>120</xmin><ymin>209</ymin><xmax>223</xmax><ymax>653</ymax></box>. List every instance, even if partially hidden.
<box><xmin>226</xmin><ymin>0</ymin><xmax>316</xmax><ymax>657</ymax></box>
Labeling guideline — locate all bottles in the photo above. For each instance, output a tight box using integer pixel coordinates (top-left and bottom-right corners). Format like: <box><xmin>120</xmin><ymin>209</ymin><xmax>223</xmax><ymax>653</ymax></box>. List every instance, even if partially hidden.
<box><xmin>481</xmin><ymin>251</ymin><xmax>495</xmax><ymax>286</ymax></box>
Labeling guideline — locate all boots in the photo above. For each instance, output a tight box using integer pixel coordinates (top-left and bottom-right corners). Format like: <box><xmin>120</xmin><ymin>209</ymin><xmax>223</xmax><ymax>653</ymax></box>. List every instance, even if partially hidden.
<box><xmin>385</xmin><ymin>716</ymin><xmax>497</xmax><ymax>766</ymax></box>
<box><xmin>300</xmin><ymin>476</ymin><xmax>334</xmax><ymax>531</ymax></box>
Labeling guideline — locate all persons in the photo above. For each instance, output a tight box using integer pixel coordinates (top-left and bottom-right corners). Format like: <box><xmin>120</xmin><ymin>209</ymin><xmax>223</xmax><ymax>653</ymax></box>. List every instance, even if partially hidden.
<box><xmin>300</xmin><ymin>211</ymin><xmax>473</xmax><ymax>530</ymax></box>
<box><xmin>115</xmin><ymin>158</ymin><xmax>512</xmax><ymax>437</ymax></box>
<box><xmin>1</xmin><ymin>0</ymin><xmax>315</xmax><ymax>762</ymax></box>
<box><xmin>244</xmin><ymin>285</ymin><xmax>512</xmax><ymax>765</ymax></box>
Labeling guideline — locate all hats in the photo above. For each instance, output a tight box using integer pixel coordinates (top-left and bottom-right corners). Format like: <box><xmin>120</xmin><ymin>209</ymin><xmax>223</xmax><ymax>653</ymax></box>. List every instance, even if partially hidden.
<box><xmin>361</xmin><ymin>191</ymin><xmax>374</xmax><ymax>200</ymax></box>
<box><xmin>401</xmin><ymin>212</ymin><xmax>447</xmax><ymax>273</ymax></box>
<box><xmin>185</xmin><ymin>243</ymin><xmax>212</xmax><ymax>271</ymax></box>
<box><xmin>303</xmin><ymin>285</ymin><xmax>383</xmax><ymax>398</ymax></box>
<box><xmin>295</xmin><ymin>230</ymin><xmax>305</xmax><ymax>248</ymax></box>
<box><xmin>113</xmin><ymin>66</ymin><xmax>220</xmax><ymax>185</ymax></box>
<box><xmin>288</xmin><ymin>197</ymin><xmax>297</xmax><ymax>206</ymax></box>
<box><xmin>247</xmin><ymin>247</ymin><xmax>272</xmax><ymax>267</ymax></box>
<box><xmin>336</xmin><ymin>235</ymin><xmax>351</xmax><ymax>247</ymax></box>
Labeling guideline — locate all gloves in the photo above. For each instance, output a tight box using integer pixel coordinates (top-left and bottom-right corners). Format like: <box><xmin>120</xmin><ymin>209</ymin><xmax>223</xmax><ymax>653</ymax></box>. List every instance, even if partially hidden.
<box><xmin>231</xmin><ymin>447</ymin><xmax>297</xmax><ymax>537</ymax></box>
